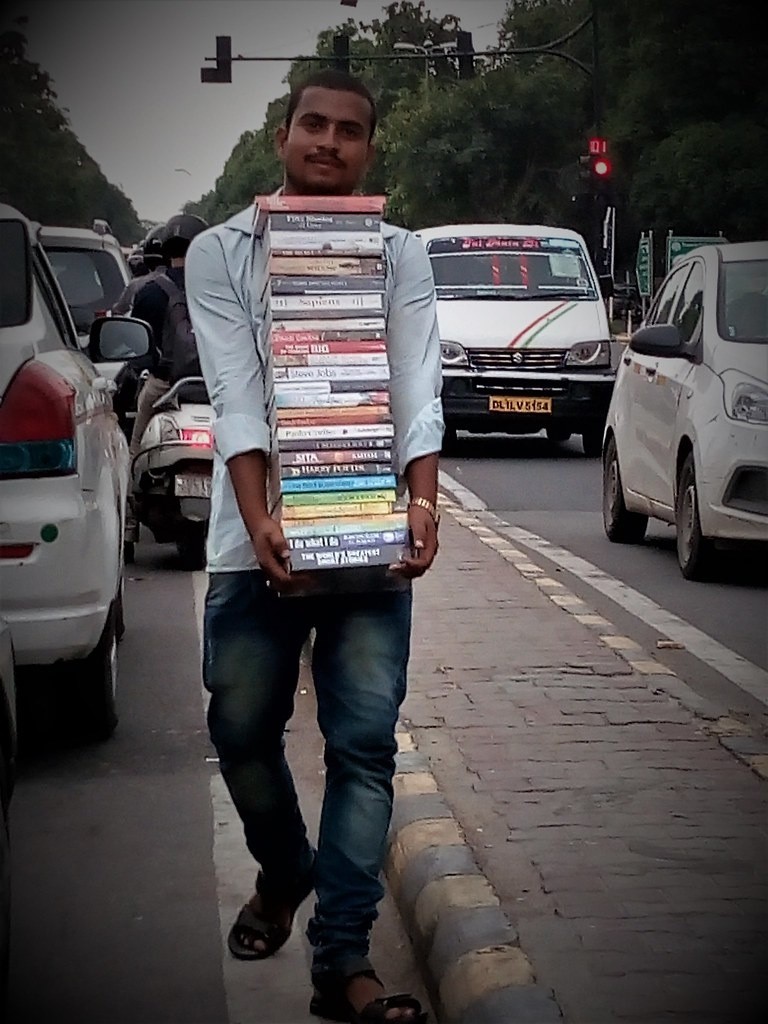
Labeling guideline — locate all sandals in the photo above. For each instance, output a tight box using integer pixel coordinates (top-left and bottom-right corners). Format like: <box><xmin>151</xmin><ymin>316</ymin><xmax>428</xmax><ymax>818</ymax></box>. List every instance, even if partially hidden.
<box><xmin>310</xmin><ymin>960</ymin><xmax>429</xmax><ymax>1024</ymax></box>
<box><xmin>228</xmin><ymin>849</ymin><xmax>316</xmax><ymax>961</ymax></box>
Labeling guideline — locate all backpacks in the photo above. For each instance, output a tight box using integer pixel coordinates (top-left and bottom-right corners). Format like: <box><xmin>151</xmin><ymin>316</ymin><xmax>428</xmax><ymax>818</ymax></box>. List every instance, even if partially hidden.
<box><xmin>153</xmin><ymin>273</ymin><xmax>210</xmax><ymax>404</ymax></box>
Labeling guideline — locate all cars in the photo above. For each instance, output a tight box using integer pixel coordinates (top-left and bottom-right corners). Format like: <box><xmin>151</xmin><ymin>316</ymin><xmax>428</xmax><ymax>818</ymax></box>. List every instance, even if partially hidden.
<box><xmin>601</xmin><ymin>243</ymin><xmax>768</xmax><ymax>583</ymax></box>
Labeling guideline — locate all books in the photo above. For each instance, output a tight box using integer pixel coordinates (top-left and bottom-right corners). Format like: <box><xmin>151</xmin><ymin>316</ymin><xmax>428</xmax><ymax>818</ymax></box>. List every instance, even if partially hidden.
<box><xmin>252</xmin><ymin>195</ymin><xmax>407</xmax><ymax>593</ymax></box>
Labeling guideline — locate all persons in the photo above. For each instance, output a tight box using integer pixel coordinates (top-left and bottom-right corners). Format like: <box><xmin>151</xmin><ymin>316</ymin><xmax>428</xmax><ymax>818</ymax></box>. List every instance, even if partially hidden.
<box><xmin>180</xmin><ymin>66</ymin><xmax>447</xmax><ymax>1024</ymax></box>
<box><xmin>119</xmin><ymin>214</ymin><xmax>211</xmax><ymax>565</ymax></box>
<box><xmin>100</xmin><ymin>222</ymin><xmax>165</xmax><ymax>445</ymax></box>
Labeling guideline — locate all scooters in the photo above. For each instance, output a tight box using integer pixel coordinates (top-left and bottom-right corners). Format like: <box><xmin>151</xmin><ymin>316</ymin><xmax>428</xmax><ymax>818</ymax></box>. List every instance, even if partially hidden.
<box><xmin>129</xmin><ymin>378</ymin><xmax>217</xmax><ymax>571</ymax></box>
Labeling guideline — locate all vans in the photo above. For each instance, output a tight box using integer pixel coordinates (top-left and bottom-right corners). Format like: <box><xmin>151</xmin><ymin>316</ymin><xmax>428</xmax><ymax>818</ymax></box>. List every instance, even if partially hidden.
<box><xmin>415</xmin><ymin>224</ymin><xmax>617</xmax><ymax>457</ymax></box>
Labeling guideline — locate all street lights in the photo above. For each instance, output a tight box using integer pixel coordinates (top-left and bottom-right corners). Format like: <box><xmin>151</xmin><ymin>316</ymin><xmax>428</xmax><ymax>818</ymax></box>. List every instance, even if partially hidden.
<box><xmin>393</xmin><ymin>42</ymin><xmax>456</xmax><ymax>105</ymax></box>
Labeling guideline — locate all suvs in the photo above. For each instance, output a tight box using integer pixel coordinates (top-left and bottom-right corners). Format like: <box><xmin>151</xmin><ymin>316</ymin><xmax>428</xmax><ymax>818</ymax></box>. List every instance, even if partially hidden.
<box><xmin>1</xmin><ymin>202</ymin><xmax>158</xmax><ymax>744</ymax></box>
<box><xmin>30</xmin><ymin>217</ymin><xmax>139</xmax><ymax>394</ymax></box>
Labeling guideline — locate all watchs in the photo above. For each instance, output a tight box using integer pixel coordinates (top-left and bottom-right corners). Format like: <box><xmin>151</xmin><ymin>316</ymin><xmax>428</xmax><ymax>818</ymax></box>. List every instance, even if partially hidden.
<box><xmin>409</xmin><ymin>498</ymin><xmax>441</xmax><ymax>529</ymax></box>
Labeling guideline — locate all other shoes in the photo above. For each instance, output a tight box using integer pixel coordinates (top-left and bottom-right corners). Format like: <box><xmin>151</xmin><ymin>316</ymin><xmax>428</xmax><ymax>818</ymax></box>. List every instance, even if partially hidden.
<box><xmin>124</xmin><ymin>541</ymin><xmax>135</xmax><ymax>564</ymax></box>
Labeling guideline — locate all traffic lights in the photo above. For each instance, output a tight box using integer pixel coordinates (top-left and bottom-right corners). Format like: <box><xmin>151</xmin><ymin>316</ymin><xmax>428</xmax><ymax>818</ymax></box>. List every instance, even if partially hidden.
<box><xmin>590</xmin><ymin>138</ymin><xmax>608</xmax><ymax>178</ymax></box>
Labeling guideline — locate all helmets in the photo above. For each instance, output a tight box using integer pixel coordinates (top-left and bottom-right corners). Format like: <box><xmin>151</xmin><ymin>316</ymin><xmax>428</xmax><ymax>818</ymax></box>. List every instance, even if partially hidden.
<box><xmin>126</xmin><ymin>214</ymin><xmax>210</xmax><ymax>278</ymax></box>
